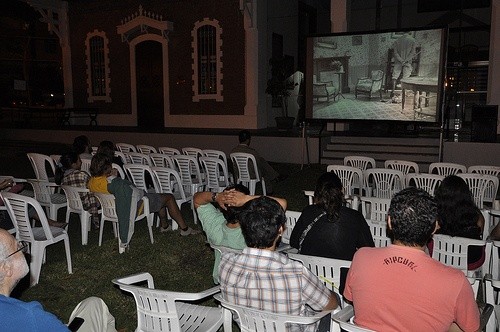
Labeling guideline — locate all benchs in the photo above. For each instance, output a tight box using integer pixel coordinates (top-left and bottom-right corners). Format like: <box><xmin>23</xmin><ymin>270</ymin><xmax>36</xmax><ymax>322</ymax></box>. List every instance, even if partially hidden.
<box><xmin>60</xmin><ymin>107</ymin><xmax>101</xmax><ymax>129</ymax></box>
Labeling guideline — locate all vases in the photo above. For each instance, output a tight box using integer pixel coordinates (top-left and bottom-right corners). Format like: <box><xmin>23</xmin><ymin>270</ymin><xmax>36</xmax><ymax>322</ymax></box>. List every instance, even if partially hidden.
<box><xmin>335</xmin><ymin>65</ymin><xmax>343</xmax><ymax>72</ymax></box>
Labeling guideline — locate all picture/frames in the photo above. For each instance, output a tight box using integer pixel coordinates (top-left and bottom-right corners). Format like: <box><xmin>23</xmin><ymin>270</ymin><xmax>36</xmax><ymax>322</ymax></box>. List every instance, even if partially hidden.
<box><xmin>314</xmin><ymin>37</ymin><xmax>337</xmax><ymax>49</ymax></box>
<box><xmin>350</xmin><ymin>35</ymin><xmax>362</xmax><ymax>46</ymax></box>
<box><xmin>391</xmin><ymin>31</ymin><xmax>417</xmax><ymax>40</ymax></box>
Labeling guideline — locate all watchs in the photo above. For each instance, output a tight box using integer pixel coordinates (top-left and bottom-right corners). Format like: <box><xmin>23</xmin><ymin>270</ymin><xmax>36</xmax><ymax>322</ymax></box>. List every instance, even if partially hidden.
<box><xmin>212</xmin><ymin>192</ymin><xmax>216</xmax><ymax>202</ymax></box>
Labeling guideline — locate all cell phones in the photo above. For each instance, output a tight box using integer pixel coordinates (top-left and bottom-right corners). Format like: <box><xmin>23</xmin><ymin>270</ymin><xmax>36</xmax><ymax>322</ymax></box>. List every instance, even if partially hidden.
<box><xmin>67</xmin><ymin>317</ymin><xmax>85</xmax><ymax>332</ymax></box>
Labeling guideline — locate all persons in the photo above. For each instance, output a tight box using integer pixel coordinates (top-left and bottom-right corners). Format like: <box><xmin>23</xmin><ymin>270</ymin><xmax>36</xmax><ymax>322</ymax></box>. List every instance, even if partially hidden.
<box><xmin>193</xmin><ymin>183</ymin><xmax>287</xmax><ymax>286</ymax></box>
<box><xmin>289</xmin><ymin>172</ymin><xmax>377</xmax><ymax>296</ymax></box>
<box><xmin>427</xmin><ymin>175</ymin><xmax>487</xmax><ymax>269</ymax></box>
<box><xmin>420</xmin><ymin>175</ymin><xmax>486</xmax><ymax>269</ymax></box>
<box><xmin>492</xmin><ymin>217</ymin><xmax>500</xmax><ymax>240</ymax></box>
<box><xmin>0</xmin><ymin>136</ymin><xmax>199</xmax><ymax>332</ymax></box>
<box><xmin>390</xmin><ymin>31</ymin><xmax>416</xmax><ymax>101</ymax></box>
<box><xmin>343</xmin><ymin>186</ymin><xmax>480</xmax><ymax>332</ymax></box>
<box><xmin>216</xmin><ymin>196</ymin><xmax>340</xmax><ymax>332</ymax></box>
<box><xmin>232</xmin><ymin>130</ymin><xmax>288</xmax><ymax>194</ymax></box>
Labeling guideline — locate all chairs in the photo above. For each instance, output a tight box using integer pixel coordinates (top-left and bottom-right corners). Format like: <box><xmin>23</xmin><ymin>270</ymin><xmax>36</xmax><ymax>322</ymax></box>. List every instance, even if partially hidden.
<box><xmin>312</xmin><ymin>80</ymin><xmax>336</xmax><ymax>106</ymax></box>
<box><xmin>413</xmin><ymin>94</ymin><xmax>437</xmax><ymax>121</ymax></box>
<box><xmin>354</xmin><ymin>70</ymin><xmax>385</xmax><ymax>101</ymax></box>
<box><xmin>0</xmin><ymin>142</ymin><xmax>500</xmax><ymax>332</ymax></box>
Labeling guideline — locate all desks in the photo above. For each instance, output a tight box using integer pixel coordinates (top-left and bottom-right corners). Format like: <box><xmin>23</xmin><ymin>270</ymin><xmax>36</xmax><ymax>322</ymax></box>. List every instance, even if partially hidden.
<box><xmin>314</xmin><ymin>55</ymin><xmax>351</xmax><ymax>93</ymax></box>
<box><xmin>401</xmin><ymin>77</ymin><xmax>438</xmax><ymax>121</ymax></box>
<box><xmin>332</xmin><ymin>71</ymin><xmax>346</xmax><ymax>102</ymax></box>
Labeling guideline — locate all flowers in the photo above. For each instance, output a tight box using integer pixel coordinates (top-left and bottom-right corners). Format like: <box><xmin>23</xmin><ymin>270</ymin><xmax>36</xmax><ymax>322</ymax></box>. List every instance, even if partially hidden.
<box><xmin>331</xmin><ymin>60</ymin><xmax>345</xmax><ymax>67</ymax></box>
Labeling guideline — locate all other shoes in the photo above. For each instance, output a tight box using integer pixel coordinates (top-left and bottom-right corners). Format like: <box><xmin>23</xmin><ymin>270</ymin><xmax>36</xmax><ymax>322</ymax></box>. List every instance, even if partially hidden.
<box><xmin>180</xmin><ymin>226</ymin><xmax>197</xmax><ymax>236</ymax></box>
<box><xmin>159</xmin><ymin>224</ymin><xmax>172</xmax><ymax>233</ymax></box>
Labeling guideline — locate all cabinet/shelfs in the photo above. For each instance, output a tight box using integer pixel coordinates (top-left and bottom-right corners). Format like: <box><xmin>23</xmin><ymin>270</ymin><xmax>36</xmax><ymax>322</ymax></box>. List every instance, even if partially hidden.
<box><xmin>385</xmin><ymin>46</ymin><xmax>422</xmax><ymax>93</ymax></box>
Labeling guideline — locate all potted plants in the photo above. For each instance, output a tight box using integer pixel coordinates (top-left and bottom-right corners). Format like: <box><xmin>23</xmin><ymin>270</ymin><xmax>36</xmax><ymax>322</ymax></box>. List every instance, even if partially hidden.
<box><xmin>264</xmin><ymin>72</ymin><xmax>299</xmax><ymax>128</ymax></box>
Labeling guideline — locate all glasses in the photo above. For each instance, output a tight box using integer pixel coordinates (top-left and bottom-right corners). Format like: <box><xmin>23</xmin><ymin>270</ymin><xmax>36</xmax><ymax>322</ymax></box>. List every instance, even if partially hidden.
<box><xmin>5</xmin><ymin>240</ymin><xmax>28</xmax><ymax>259</ymax></box>
